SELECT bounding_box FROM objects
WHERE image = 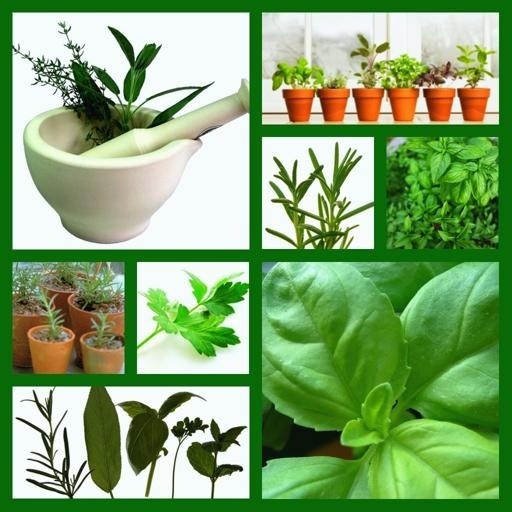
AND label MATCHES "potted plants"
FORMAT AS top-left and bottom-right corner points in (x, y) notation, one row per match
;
(12, 262), (125, 373)
(272, 32), (496, 122)
